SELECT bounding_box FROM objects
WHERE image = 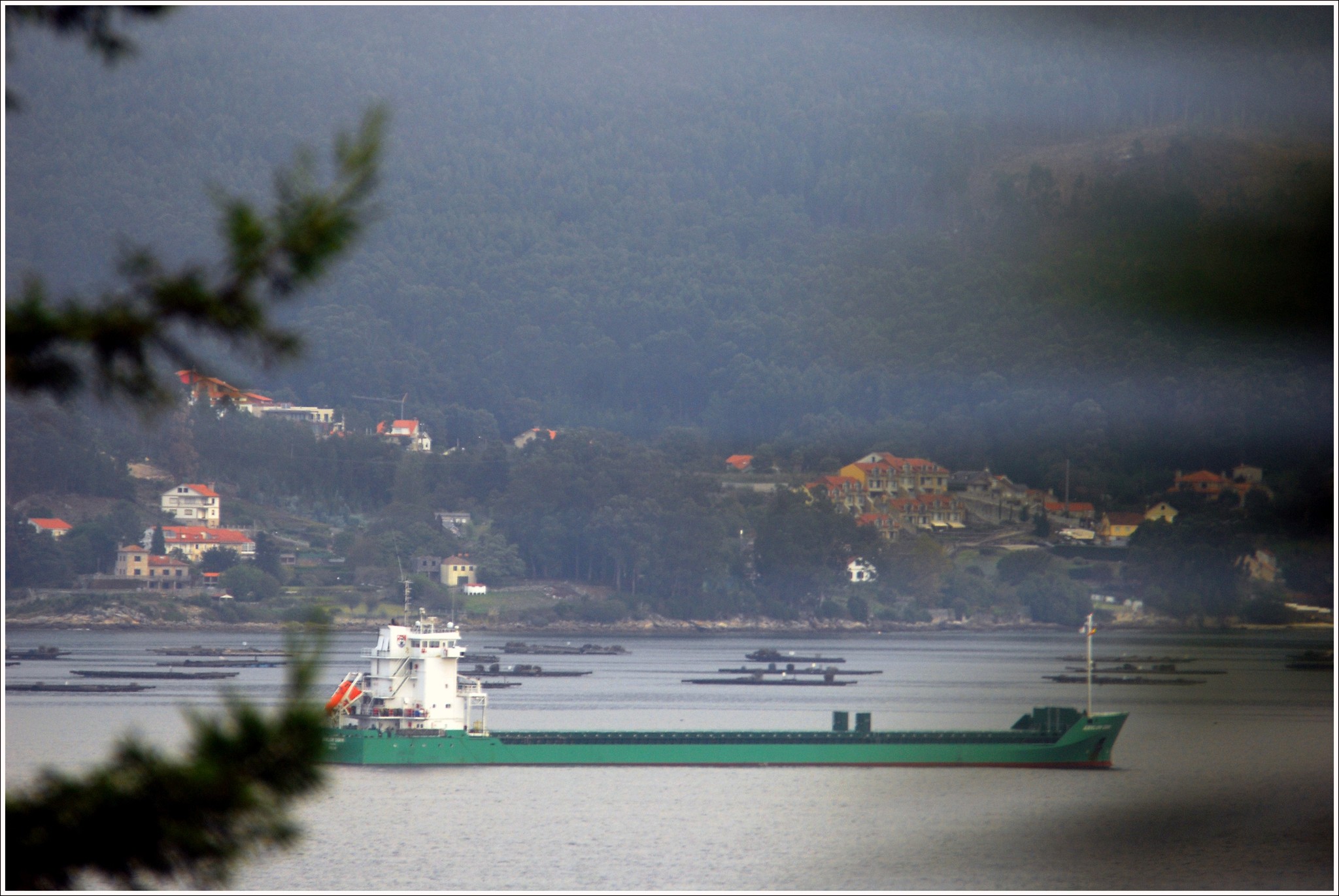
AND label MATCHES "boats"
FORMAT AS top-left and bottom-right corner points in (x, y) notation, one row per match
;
(454, 679), (523, 689)
(1036, 672), (1210, 688)
(6, 674), (159, 693)
(456, 651), (501, 662)
(450, 662), (595, 678)
(746, 646), (848, 664)
(141, 642), (295, 656)
(1282, 651), (1333, 669)
(6, 647), (71, 660)
(485, 638), (630, 656)
(1064, 662), (1231, 676)
(717, 662), (885, 676)
(681, 672), (860, 685)
(1059, 652), (1203, 664)
(153, 656), (293, 668)
(72, 666), (242, 681)
(315, 579), (1130, 770)
